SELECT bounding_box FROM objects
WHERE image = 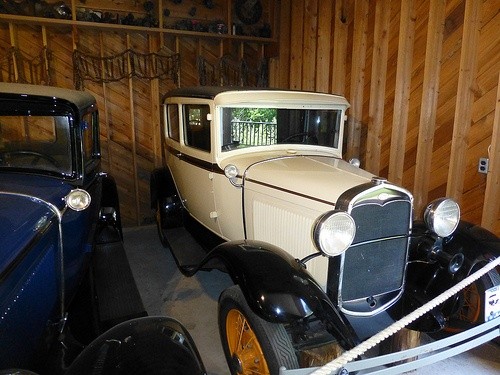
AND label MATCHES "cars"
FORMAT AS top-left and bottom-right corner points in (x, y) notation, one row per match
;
(147, 85), (499, 375)
(1, 81), (206, 375)
